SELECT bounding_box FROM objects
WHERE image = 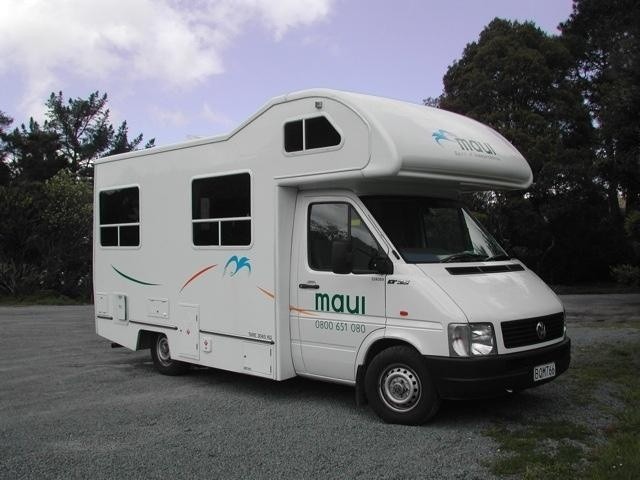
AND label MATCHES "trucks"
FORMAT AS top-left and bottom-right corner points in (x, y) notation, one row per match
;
(90, 90), (572, 426)
